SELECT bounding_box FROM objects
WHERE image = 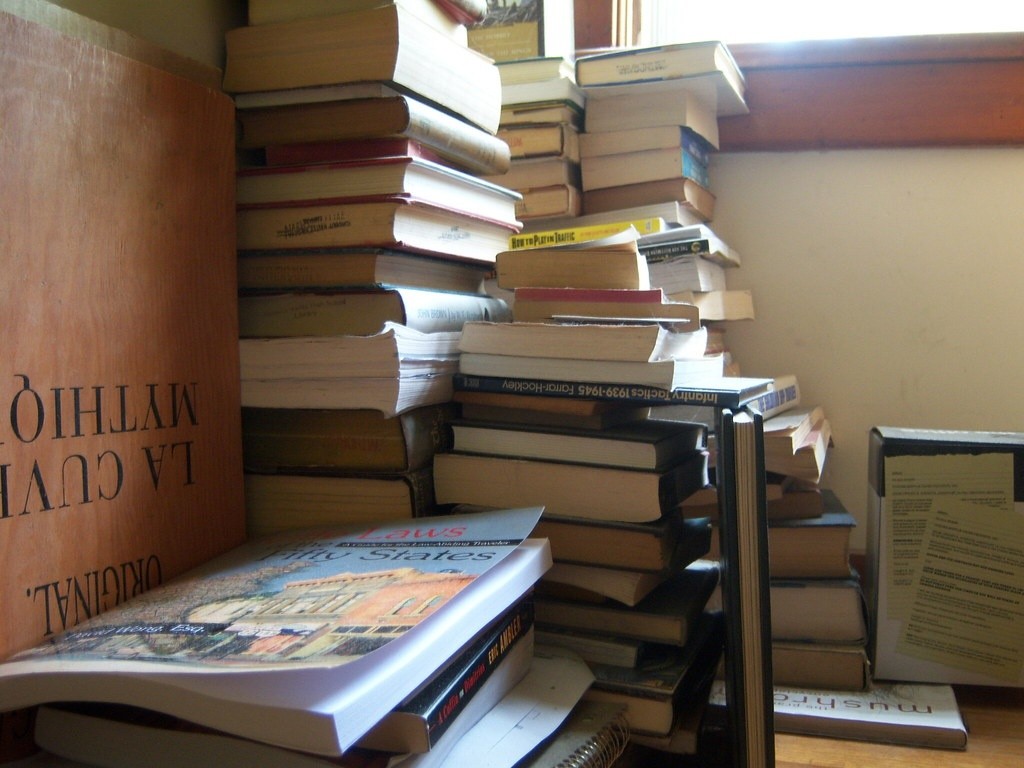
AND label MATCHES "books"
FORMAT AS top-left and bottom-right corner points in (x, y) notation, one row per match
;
(1, 1), (970, 767)
